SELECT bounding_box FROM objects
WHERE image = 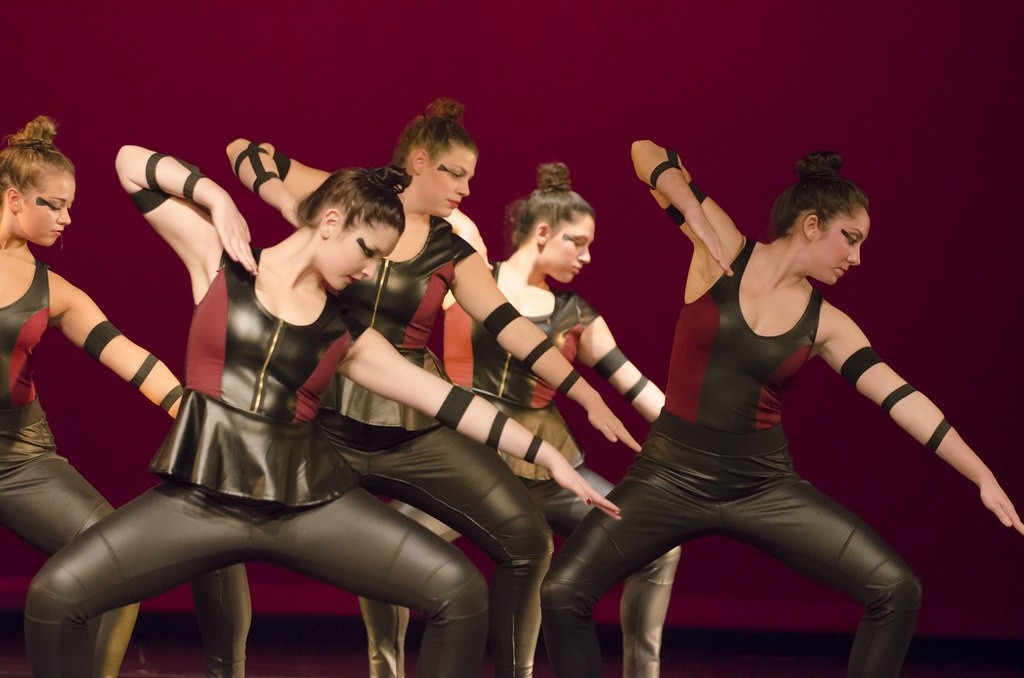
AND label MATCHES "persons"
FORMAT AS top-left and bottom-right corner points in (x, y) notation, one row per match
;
(0, 115), (184, 678)
(24, 145), (624, 677)
(354, 162), (681, 678)
(190, 98), (644, 678)
(539, 141), (1024, 678)
